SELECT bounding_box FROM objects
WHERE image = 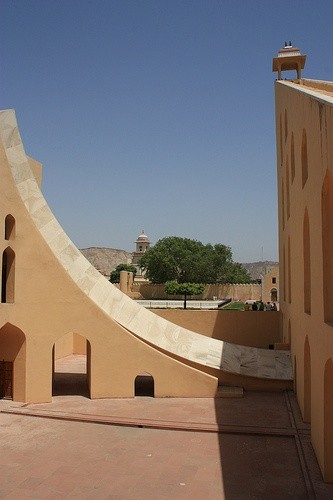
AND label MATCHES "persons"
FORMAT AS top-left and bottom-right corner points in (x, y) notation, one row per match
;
(272, 303), (277, 311)
(252, 302), (257, 310)
(266, 302), (272, 311)
(245, 302), (250, 311)
(258, 302), (264, 311)
